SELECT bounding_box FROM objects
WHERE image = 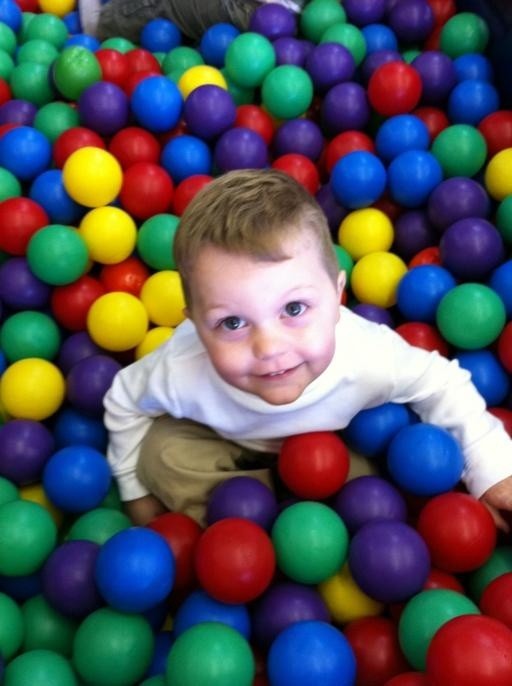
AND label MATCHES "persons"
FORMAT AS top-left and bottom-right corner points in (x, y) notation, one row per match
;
(106, 168), (512, 529)
